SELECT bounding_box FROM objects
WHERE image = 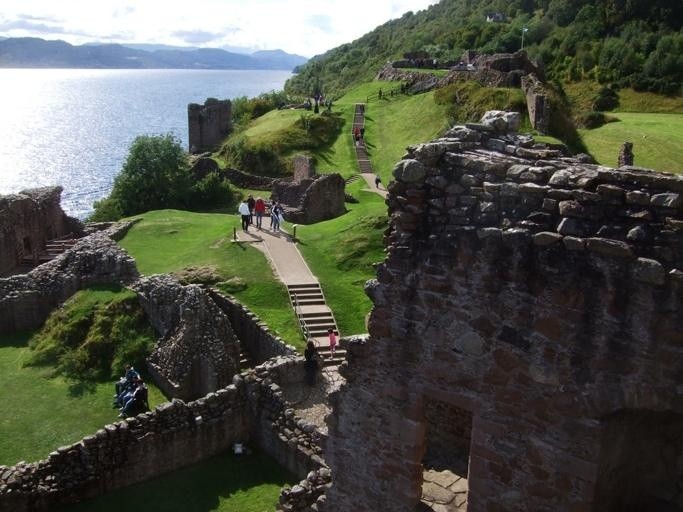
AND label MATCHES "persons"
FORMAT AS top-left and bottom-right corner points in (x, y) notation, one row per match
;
(375, 173), (381, 188)
(238, 193), (285, 233)
(112, 364), (148, 418)
(354, 126), (365, 147)
(304, 339), (316, 386)
(328, 327), (337, 360)
